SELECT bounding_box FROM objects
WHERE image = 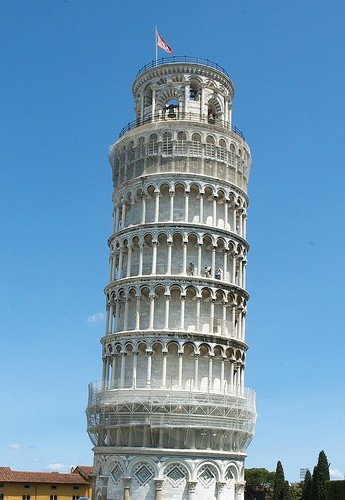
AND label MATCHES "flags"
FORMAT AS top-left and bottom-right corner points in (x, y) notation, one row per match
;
(157, 34), (172, 54)
